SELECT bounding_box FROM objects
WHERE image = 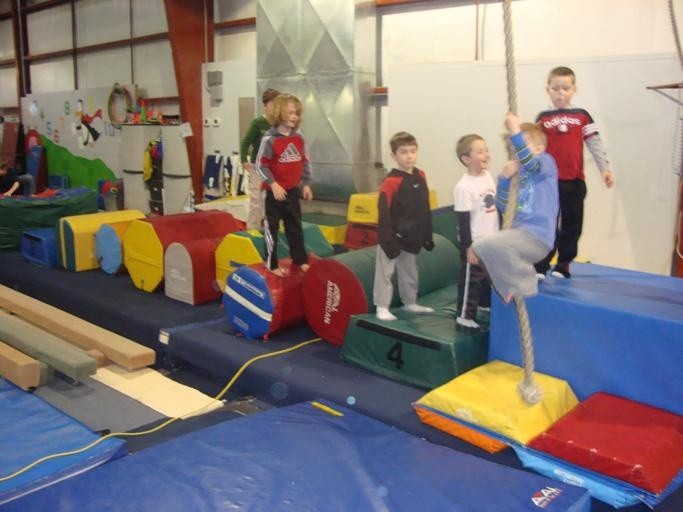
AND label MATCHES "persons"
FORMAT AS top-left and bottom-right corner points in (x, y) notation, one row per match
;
(533, 64), (615, 282)
(256, 92), (314, 278)
(0, 164), (36, 199)
(238, 86), (282, 232)
(466, 109), (560, 305)
(449, 131), (503, 332)
(368, 129), (438, 322)
